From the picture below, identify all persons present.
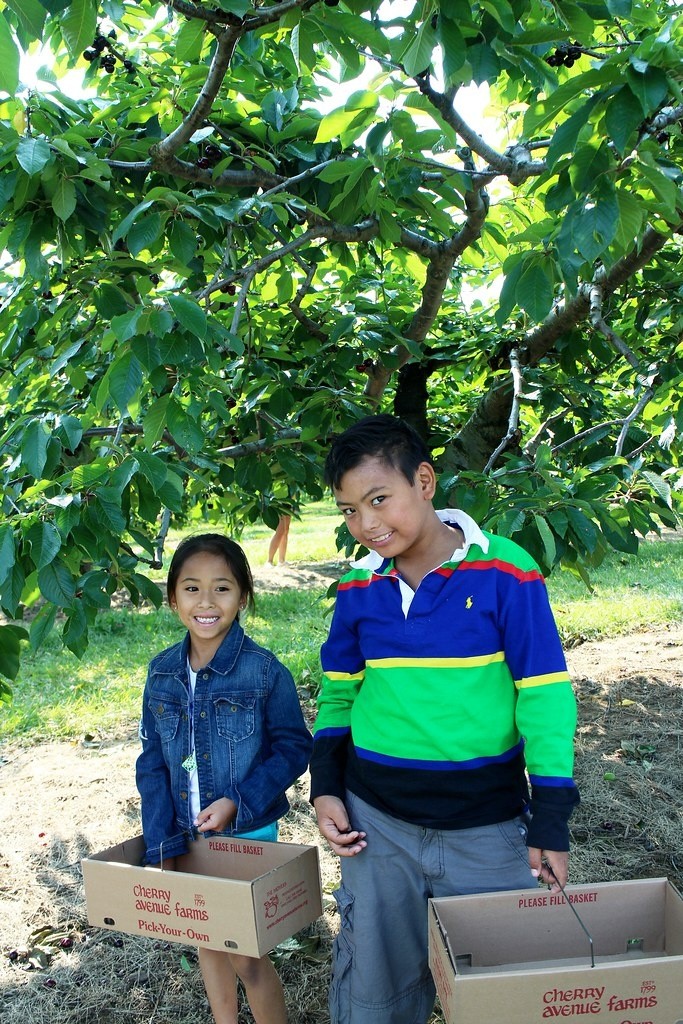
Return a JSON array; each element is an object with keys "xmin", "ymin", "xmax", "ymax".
[
  {"xmin": 135, "ymin": 533, "xmax": 310, "ymax": 1024},
  {"xmin": 268, "ymin": 482, "xmax": 300, "ymax": 567},
  {"xmin": 310, "ymin": 415, "xmax": 581, "ymax": 1023}
]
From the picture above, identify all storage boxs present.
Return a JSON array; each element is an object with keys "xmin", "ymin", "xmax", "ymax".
[
  {"xmin": 428, "ymin": 877, "xmax": 683, "ymax": 1023},
  {"xmin": 81, "ymin": 834, "xmax": 325, "ymax": 959}
]
